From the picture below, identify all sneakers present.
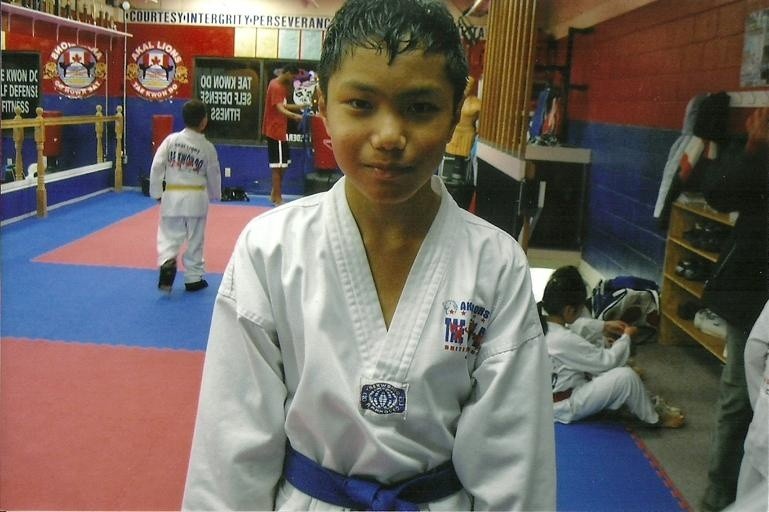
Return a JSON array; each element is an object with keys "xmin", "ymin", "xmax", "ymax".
[
  {"xmin": 159, "ymin": 262, "xmax": 176, "ymax": 291},
  {"xmin": 650, "ymin": 395, "xmax": 684, "ymax": 427},
  {"xmin": 703, "ymin": 479, "xmax": 738, "ymax": 509},
  {"xmin": 674, "ymin": 220, "xmax": 727, "ymax": 340},
  {"xmin": 185, "ymin": 279, "xmax": 209, "ymax": 291}
]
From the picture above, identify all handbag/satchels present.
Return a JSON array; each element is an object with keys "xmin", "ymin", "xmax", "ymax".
[
  {"xmin": 303, "ymin": 147, "xmax": 316, "ymax": 174},
  {"xmin": 585, "ymin": 275, "xmax": 660, "ymax": 345}
]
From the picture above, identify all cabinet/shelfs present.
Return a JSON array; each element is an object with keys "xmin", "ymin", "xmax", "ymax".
[{"xmin": 659, "ymin": 190, "xmax": 737, "ymax": 372}]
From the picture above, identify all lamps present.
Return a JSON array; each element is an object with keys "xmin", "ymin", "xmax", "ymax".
[{"xmin": 117, "ymin": 0, "xmax": 131, "ymax": 12}]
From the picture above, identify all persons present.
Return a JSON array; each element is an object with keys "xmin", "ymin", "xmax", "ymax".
[
  {"xmin": 147, "ymin": 98, "xmax": 223, "ymax": 293},
  {"xmin": 443, "ymin": 74, "xmax": 482, "ymax": 158},
  {"xmin": 700, "ymin": 106, "xmax": 769, "ymax": 510},
  {"xmin": 58, "ymin": 60, "xmax": 173, "ymax": 81},
  {"xmin": 178, "ymin": 0, "xmax": 558, "ymax": 511},
  {"xmin": 722, "ymin": 297, "xmax": 769, "ymax": 511},
  {"xmin": 261, "ymin": 63, "xmax": 308, "ymax": 205},
  {"xmin": 536, "ymin": 265, "xmax": 686, "ymax": 430}
]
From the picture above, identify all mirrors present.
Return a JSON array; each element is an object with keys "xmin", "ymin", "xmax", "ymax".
[{"xmin": 1, "ymin": 8, "xmax": 130, "ymax": 195}]
